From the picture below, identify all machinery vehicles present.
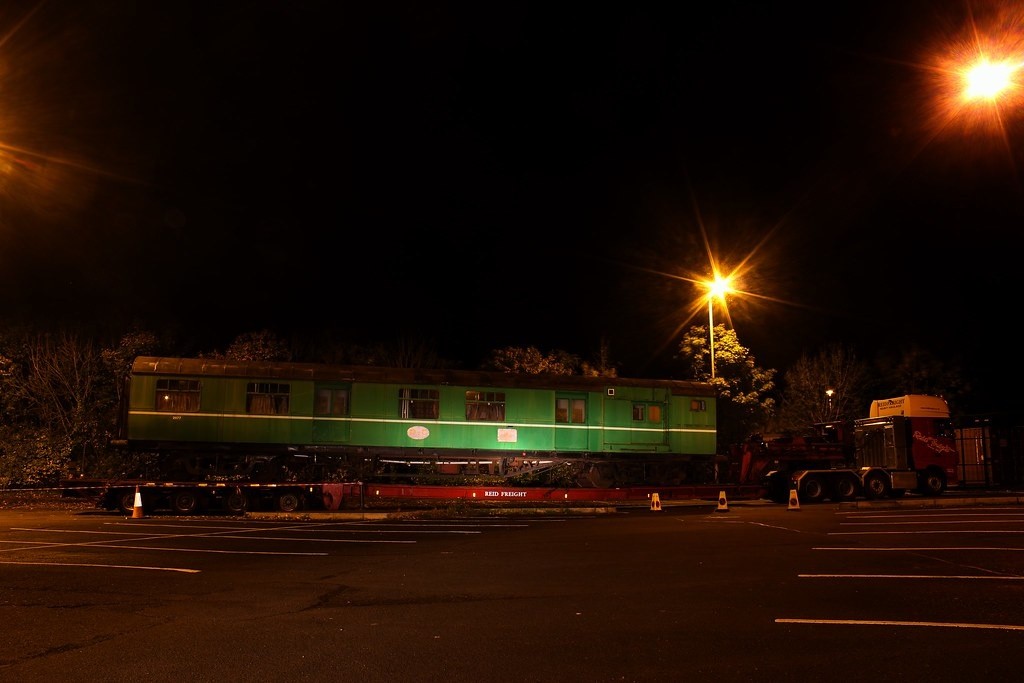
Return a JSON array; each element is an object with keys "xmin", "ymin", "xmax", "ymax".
[{"xmin": 762, "ymin": 421, "xmax": 853, "ymax": 467}]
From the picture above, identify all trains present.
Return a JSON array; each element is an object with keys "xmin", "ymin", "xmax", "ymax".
[{"xmin": 126, "ymin": 355, "xmax": 718, "ymax": 485}]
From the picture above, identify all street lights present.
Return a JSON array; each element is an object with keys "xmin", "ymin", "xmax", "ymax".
[{"xmin": 705, "ymin": 274, "xmax": 734, "ymax": 378}]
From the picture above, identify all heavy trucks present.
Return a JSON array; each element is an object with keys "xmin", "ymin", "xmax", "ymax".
[{"xmin": 766, "ymin": 393, "xmax": 960, "ymax": 499}]
